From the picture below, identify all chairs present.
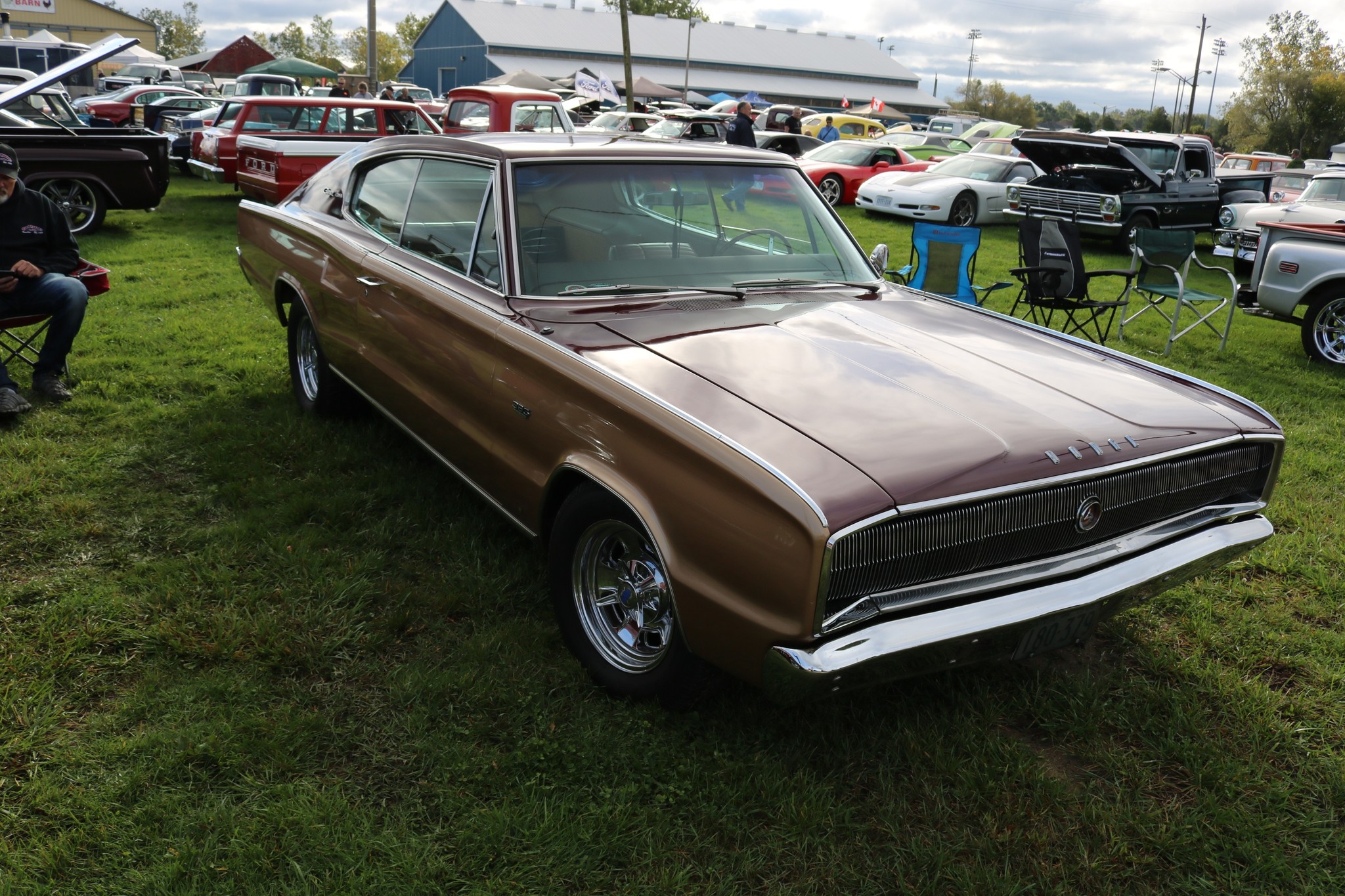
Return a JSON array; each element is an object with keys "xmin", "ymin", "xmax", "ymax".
[
  {"xmin": 879, "ymin": 154, "xmax": 893, "ymax": 165},
  {"xmin": 884, "ymin": 220, "xmax": 1013, "ymax": 307},
  {"xmin": 0, "ymin": 258, "xmax": 111, "ymax": 386},
  {"xmin": 1009, "ymin": 220, "xmax": 1139, "ymax": 348},
  {"xmin": 391, "ymin": 222, "xmax": 483, "ymax": 253},
  {"xmin": 432, "ymin": 250, "xmax": 499, "ymax": 280},
  {"xmin": 608, "ymin": 242, "xmax": 697, "ymax": 260},
  {"xmin": 1118, "ymin": 226, "xmax": 1238, "ymax": 359}
]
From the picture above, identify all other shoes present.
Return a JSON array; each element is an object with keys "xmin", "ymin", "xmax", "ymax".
[
  {"xmin": 32, "ymin": 371, "xmax": 73, "ymax": 402},
  {"xmin": 0, "ymin": 388, "xmax": 31, "ymax": 414}
]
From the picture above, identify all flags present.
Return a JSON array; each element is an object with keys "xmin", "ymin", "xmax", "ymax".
[
  {"xmin": 871, "ymin": 96, "xmax": 885, "ymax": 112},
  {"xmin": 575, "ymin": 71, "xmax": 604, "ymax": 101},
  {"xmin": 840, "ymin": 95, "xmax": 849, "ymax": 108},
  {"xmin": 599, "ymin": 70, "xmax": 622, "ymax": 106}
]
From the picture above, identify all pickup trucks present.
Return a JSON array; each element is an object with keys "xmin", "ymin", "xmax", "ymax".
[
  {"xmin": 1000, "ymin": 132, "xmax": 1277, "ymax": 257},
  {"xmin": 1234, "ymin": 221, "xmax": 1344, "ymax": 368},
  {"xmin": 238, "ymin": 87, "xmax": 584, "ymax": 207}
]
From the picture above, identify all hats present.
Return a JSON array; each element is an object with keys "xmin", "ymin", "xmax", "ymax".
[
  {"xmin": 1288, "ymin": 149, "xmax": 1299, "ymax": 155},
  {"xmin": 0, "ymin": 144, "xmax": 20, "ymax": 179},
  {"xmin": 386, "ymin": 86, "xmax": 393, "ymax": 92}
]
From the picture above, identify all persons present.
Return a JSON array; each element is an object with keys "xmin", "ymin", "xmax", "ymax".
[
  {"xmin": 785, "ymin": 106, "xmax": 840, "ymax": 143},
  {"xmin": 98, "ymin": 68, "xmax": 116, "ymax": 78},
  {"xmin": 1285, "ymin": 149, "xmax": 1305, "ymax": 188},
  {"xmin": 0, "ymin": 143, "xmax": 89, "ymax": 418},
  {"xmin": 721, "ymin": 101, "xmax": 755, "ymax": 211},
  {"xmin": 295, "ymin": 75, "xmax": 415, "ymax": 129},
  {"xmin": 845, "ymin": 110, "xmax": 888, "ymax": 128}
]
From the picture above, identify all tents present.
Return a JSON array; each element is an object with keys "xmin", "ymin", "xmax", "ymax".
[
  {"xmin": 841, "ymin": 103, "xmax": 911, "ymax": 124},
  {"xmin": 479, "ymin": 69, "xmax": 773, "ymax": 111},
  {"xmin": 26, "ymin": 28, "xmax": 65, "ymax": 43},
  {"xmin": 88, "ymin": 33, "xmax": 165, "ymax": 65},
  {"xmin": 244, "ymin": 56, "xmax": 338, "ymax": 87}
]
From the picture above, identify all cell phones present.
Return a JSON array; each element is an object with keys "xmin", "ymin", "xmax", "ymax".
[{"xmin": 0, "ymin": 271, "xmax": 16, "ymax": 279}]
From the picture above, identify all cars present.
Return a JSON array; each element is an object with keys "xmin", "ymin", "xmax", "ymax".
[
  {"xmin": 1213, "ymin": 146, "xmax": 1344, "ymax": 260},
  {"xmin": 0, "ymin": 38, "xmax": 1045, "ymax": 235},
  {"xmin": 235, "ymin": 133, "xmax": 1287, "ymax": 696}
]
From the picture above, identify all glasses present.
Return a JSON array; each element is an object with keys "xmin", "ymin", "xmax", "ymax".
[
  {"xmin": 796, "ymin": 112, "xmax": 802, "ymax": 114},
  {"xmin": 338, "ymin": 82, "xmax": 343, "ymax": 85},
  {"xmin": 827, "ymin": 122, "xmax": 831, "ymax": 123}
]
